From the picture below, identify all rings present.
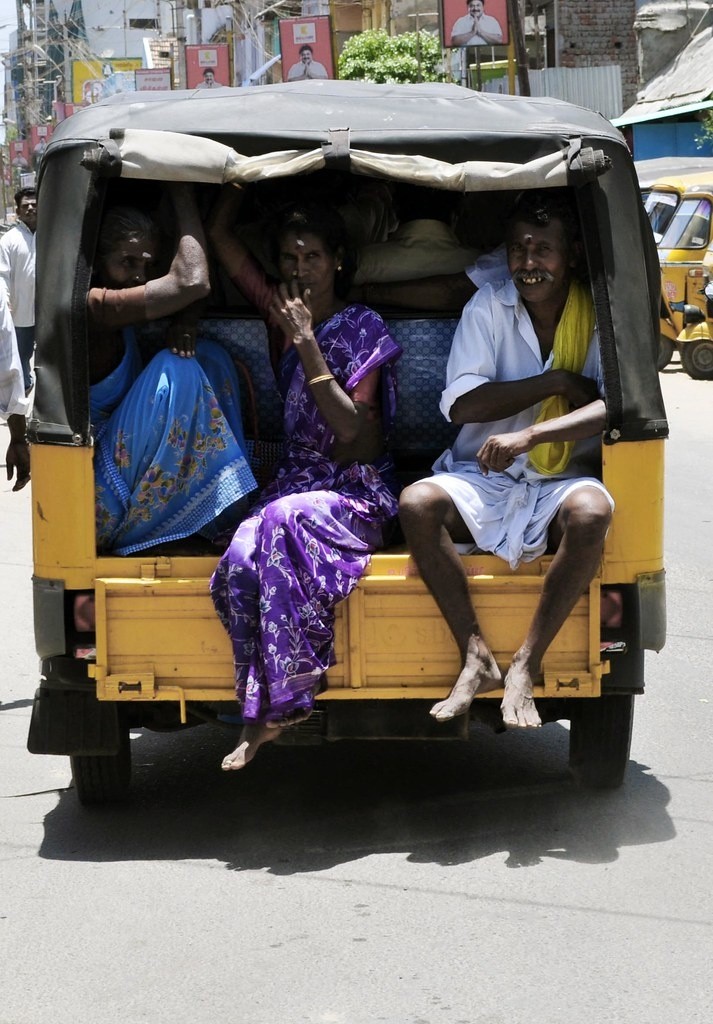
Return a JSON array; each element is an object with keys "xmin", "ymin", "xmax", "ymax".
[{"xmin": 184, "ymin": 333, "xmax": 192, "ymax": 337}]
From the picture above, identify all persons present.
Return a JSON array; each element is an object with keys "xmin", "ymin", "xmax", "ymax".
[
  {"xmin": 12, "ymin": 151, "xmax": 28, "ymax": 165},
  {"xmin": 85, "ymin": 181, "xmax": 259, "ymax": 557},
  {"xmin": 33, "ymin": 136, "xmax": 47, "ymax": 153},
  {"xmin": 344, "ymin": 191, "xmax": 616, "ymax": 730},
  {"xmin": 0, "ymin": 185, "xmax": 38, "ymax": 398},
  {"xmin": 353, "ymin": 182, "xmax": 486, "ymax": 285},
  {"xmin": 450, "ymin": 0, "xmax": 503, "ymax": 46},
  {"xmin": 195, "ymin": 69, "xmax": 223, "ymax": 89},
  {"xmin": 288, "ymin": 45, "xmax": 328, "ymax": 82},
  {"xmin": 209, "ymin": 181, "xmax": 406, "ymax": 771},
  {"xmin": 0, "ymin": 289, "xmax": 32, "ymax": 492}
]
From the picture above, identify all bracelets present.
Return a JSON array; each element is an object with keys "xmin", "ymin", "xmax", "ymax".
[{"xmin": 306, "ymin": 374, "xmax": 335, "ymax": 386}]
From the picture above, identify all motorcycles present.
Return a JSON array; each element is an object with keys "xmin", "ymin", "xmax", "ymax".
[
  {"xmin": 644, "ymin": 169, "xmax": 713, "ymax": 379},
  {"xmin": 24, "ymin": 79, "xmax": 670, "ymax": 810}
]
